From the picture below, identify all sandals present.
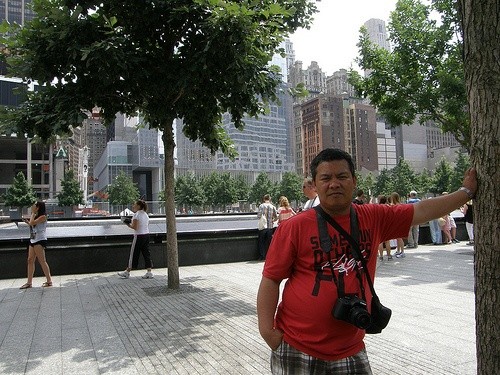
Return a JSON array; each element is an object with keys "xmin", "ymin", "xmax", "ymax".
[
  {"xmin": 42, "ymin": 282, "xmax": 52, "ymax": 287},
  {"xmin": 19, "ymin": 283, "xmax": 32, "ymax": 289}
]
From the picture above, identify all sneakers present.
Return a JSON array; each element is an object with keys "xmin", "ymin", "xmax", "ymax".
[
  {"xmin": 118, "ymin": 270, "xmax": 130, "ymax": 279},
  {"xmin": 141, "ymin": 272, "xmax": 152, "ymax": 279}
]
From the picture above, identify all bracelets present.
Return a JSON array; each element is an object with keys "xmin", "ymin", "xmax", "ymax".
[{"xmin": 459, "ymin": 188, "xmax": 474, "ymax": 200}]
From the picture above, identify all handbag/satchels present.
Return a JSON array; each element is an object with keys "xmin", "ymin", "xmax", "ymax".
[{"xmin": 258, "ymin": 202, "xmax": 267, "ymax": 230}]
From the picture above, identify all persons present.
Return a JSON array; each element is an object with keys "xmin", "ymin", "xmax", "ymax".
[
  {"xmin": 118, "ymin": 200, "xmax": 154, "ymax": 278},
  {"xmin": 176, "ymin": 208, "xmax": 194, "ymax": 216},
  {"xmin": 255, "ymin": 194, "xmax": 278, "ymax": 261},
  {"xmin": 277, "ymin": 196, "xmax": 297, "ymax": 224},
  {"xmin": 292, "ymin": 205, "xmax": 304, "ymax": 214},
  {"xmin": 302, "ymin": 177, "xmax": 321, "ymax": 211},
  {"xmin": 352, "ymin": 190, "xmax": 474, "ymax": 260},
  {"xmin": 20, "ymin": 201, "xmax": 52, "ymax": 288},
  {"xmin": 256, "ymin": 148, "xmax": 478, "ymax": 375}
]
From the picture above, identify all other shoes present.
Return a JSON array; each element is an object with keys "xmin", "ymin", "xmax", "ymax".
[
  {"xmin": 466, "ymin": 241, "xmax": 474, "ymax": 246},
  {"xmin": 452, "ymin": 238, "xmax": 460, "ymax": 244},
  {"xmin": 443, "ymin": 241, "xmax": 448, "ymax": 244},
  {"xmin": 447, "ymin": 241, "xmax": 451, "ymax": 244},
  {"xmin": 433, "ymin": 242, "xmax": 442, "ymax": 245},
  {"xmin": 392, "ymin": 251, "xmax": 402, "ymax": 258},
  {"xmin": 400, "ymin": 252, "xmax": 405, "ymax": 258},
  {"xmin": 406, "ymin": 243, "xmax": 414, "ymax": 248},
  {"xmin": 388, "ymin": 256, "xmax": 393, "ymax": 261},
  {"xmin": 377, "ymin": 256, "xmax": 383, "ymax": 261},
  {"xmin": 414, "ymin": 243, "xmax": 418, "ymax": 248}
]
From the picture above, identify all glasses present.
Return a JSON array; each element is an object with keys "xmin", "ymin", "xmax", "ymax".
[{"xmin": 303, "ymin": 185, "xmax": 314, "ymax": 189}]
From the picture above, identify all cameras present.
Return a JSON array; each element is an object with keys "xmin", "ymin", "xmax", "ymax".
[{"xmin": 333, "ymin": 296, "xmax": 371, "ymax": 328}]
